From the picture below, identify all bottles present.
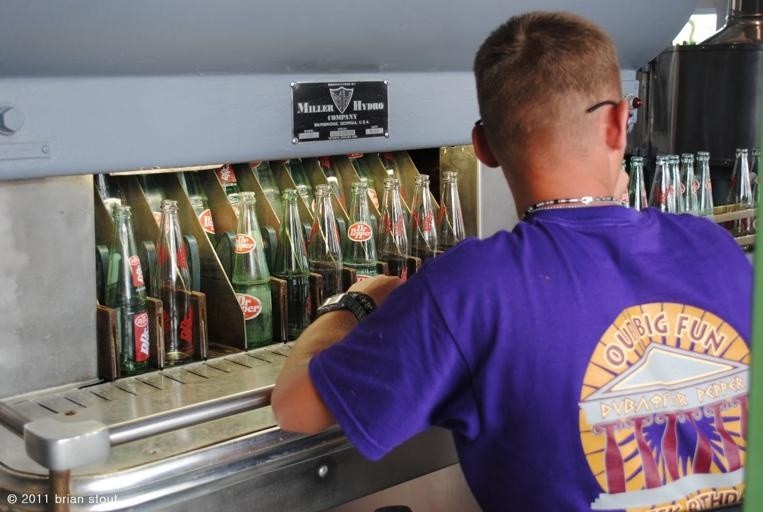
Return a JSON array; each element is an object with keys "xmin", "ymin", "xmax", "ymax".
[
  {"xmin": 377, "ymin": 152, "xmax": 406, "ymax": 186},
  {"xmin": 105, "ymin": 201, "xmax": 152, "ymax": 373},
  {"xmin": 184, "ymin": 170, "xmax": 221, "ymax": 244},
  {"xmin": 319, "ymin": 160, "xmax": 344, "ymax": 199},
  {"xmin": 680, "ymin": 152, "xmax": 698, "ymax": 212},
  {"xmin": 619, "ymin": 160, "xmax": 631, "ymax": 208},
  {"xmin": 155, "ymin": 197, "xmax": 198, "ymax": 362},
  {"xmin": 381, "ymin": 177, "xmax": 410, "ymax": 279},
  {"xmin": 277, "ymin": 187, "xmax": 314, "ymax": 342},
  {"xmin": 235, "ymin": 192, "xmax": 275, "ymax": 344},
  {"xmin": 727, "ymin": 147, "xmax": 753, "ymax": 251},
  {"xmin": 350, "ymin": 182, "xmax": 380, "ymax": 280},
  {"xmin": 215, "ymin": 166, "xmax": 241, "ymax": 198},
  {"xmin": 695, "ymin": 151, "xmax": 714, "ymax": 214},
  {"xmin": 437, "ymin": 171, "xmax": 467, "ymax": 252},
  {"xmin": 98, "ymin": 173, "xmax": 138, "ymax": 235},
  {"xmin": 649, "ymin": 156, "xmax": 671, "ymax": 212},
  {"xmin": 144, "ymin": 172, "xmax": 181, "ymax": 226},
  {"xmin": 669, "ymin": 155, "xmax": 682, "ymax": 213},
  {"xmin": 629, "ymin": 156, "xmax": 650, "ymax": 210},
  {"xmin": 286, "ymin": 160, "xmax": 313, "ymax": 205},
  {"xmin": 313, "ymin": 183, "xmax": 345, "ymax": 305},
  {"xmin": 348, "ymin": 154, "xmax": 375, "ymax": 189},
  {"xmin": 412, "ymin": 175, "xmax": 435, "ymax": 265},
  {"xmin": 249, "ymin": 160, "xmax": 283, "ymax": 218}
]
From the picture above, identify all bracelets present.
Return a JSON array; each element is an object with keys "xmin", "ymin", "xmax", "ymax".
[{"xmin": 348, "ymin": 292, "xmax": 378, "ymax": 315}]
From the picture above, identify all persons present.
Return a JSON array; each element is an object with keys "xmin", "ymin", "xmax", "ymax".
[{"xmin": 268, "ymin": 10, "xmax": 754, "ymax": 512}]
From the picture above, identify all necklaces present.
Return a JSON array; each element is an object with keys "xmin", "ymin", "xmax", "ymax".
[{"xmin": 519, "ymin": 196, "xmax": 626, "ymax": 215}]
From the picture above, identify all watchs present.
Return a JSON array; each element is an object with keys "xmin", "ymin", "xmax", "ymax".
[{"xmin": 315, "ymin": 293, "xmax": 368, "ymax": 324}]
